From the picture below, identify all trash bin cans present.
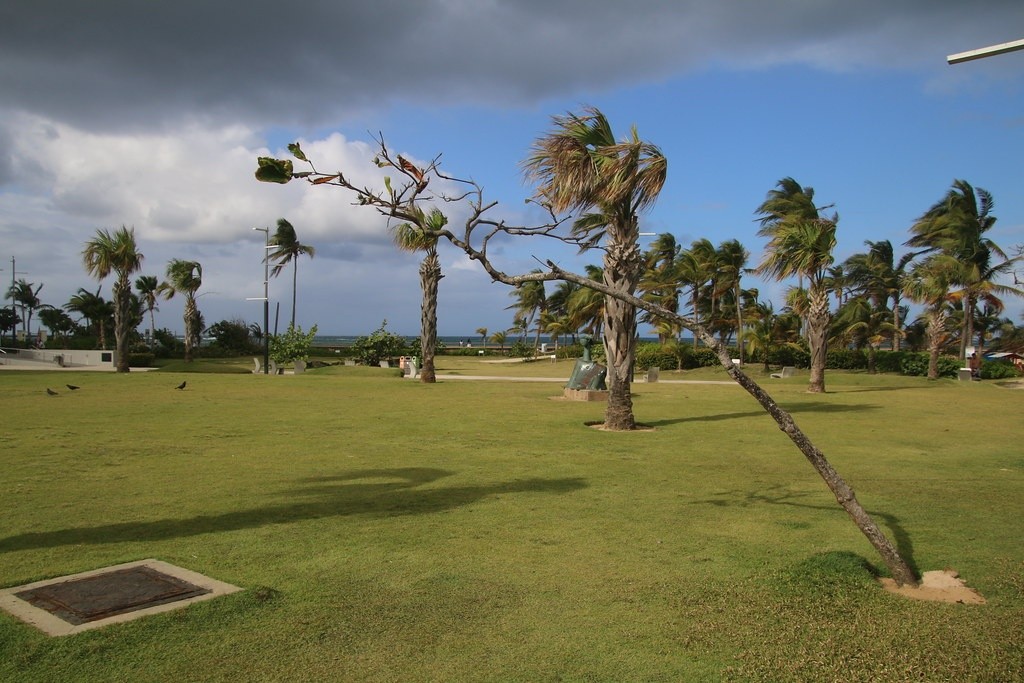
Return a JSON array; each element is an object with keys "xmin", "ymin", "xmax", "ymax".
[
  {"xmin": 399, "ymin": 356, "xmax": 419, "ymax": 375},
  {"xmin": 541, "ymin": 343, "xmax": 547, "ymax": 352}
]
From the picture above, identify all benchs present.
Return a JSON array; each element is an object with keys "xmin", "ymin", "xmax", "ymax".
[{"xmin": 771, "ymin": 367, "xmax": 795, "ymax": 379}]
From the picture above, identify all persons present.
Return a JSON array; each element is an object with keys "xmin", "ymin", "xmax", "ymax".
[
  {"xmin": 467, "ymin": 339, "xmax": 471, "ymax": 347},
  {"xmin": 459, "ymin": 340, "xmax": 463, "ymax": 346},
  {"xmin": 37, "ymin": 338, "xmax": 45, "ymax": 349}
]
